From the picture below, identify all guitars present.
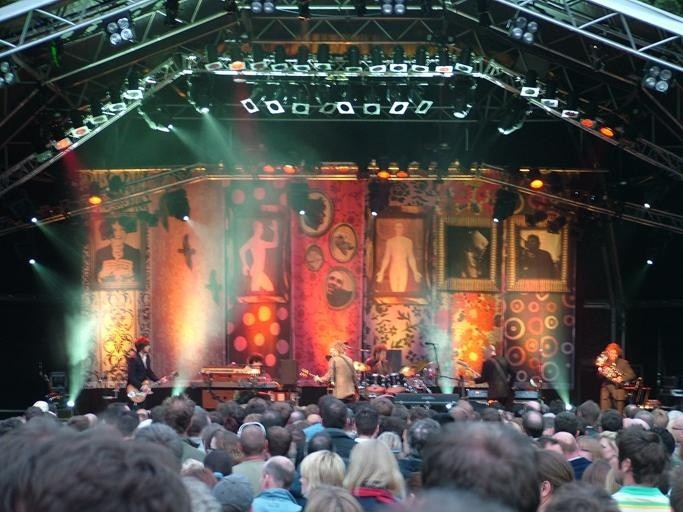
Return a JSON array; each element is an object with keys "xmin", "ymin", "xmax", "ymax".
[
  {"xmin": 127, "ymin": 371, "xmax": 180, "ymax": 404},
  {"xmin": 300, "ymin": 368, "xmax": 359, "ymax": 404}
]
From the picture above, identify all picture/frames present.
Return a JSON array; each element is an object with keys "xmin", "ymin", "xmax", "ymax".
[
  {"xmin": 89, "ymin": 210, "xmax": 148, "ymax": 290},
  {"xmin": 435, "ymin": 214, "xmax": 497, "ymax": 292},
  {"xmin": 505, "ymin": 214, "xmax": 568, "ymax": 294}
]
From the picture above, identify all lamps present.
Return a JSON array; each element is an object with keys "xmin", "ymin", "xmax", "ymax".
[
  {"xmin": 497, "ymin": 70, "xmax": 639, "ymax": 139},
  {"xmin": 366, "ymin": 178, "xmax": 392, "ymax": 216},
  {"xmin": 0, "ymin": 62, "xmax": 19, "ymax": 89},
  {"xmin": 508, "ymin": 15, "xmax": 539, "ymax": 42},
  {"xmin": 31, "ymin": 66, "xmax": 211, "ymax": 161},
  {"xmin": 524, "ymin": 199, "xmax": 568, "ymax": 236},
  {"xmin": 234, "ymin": 78, "xmax": 477, "ymax": 118},
  {"xmin": 186, "ymin": 40, "xmax": 474, "ymax": 78},
  {"xmin": 163, "ymin": 0, "xmax": 179, "ymax": 26},
  {"xmin": 488, "ymin": 190, "xmax": 521, "ymax": 225},
  {"xmin": 158, "ymin": 188, "xmax": 194, "ymax": 226},
  {"xmin": 252, "ymin": 0, "xmax": 437, "ymax": 20},
  {"xmin": 105, "ymin": 11, "xmax": 136, "ymax": 48},
  {"xmin": 643, "ymin": 65, "xmax": 674, "ymax": 93},
  {"xmin": 284, "ymin": 182, "xmax": 312, "ymax": 215},
  {"xmin": 96, "ymin": 200, "xmax": 158, "ymax": 241},
  {"xmin": 86, "ymin": 179, "xmax": 104, "ymax": 206}
]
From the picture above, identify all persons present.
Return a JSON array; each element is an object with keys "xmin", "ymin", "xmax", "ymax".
[
  {"xmin": 94, "ymin": 217, "xmax": 141, "ymax": 288},
  {"xmin": 376, "ymin": 222, "xmax": 423, "ymax": 293},
  {"xmin": 524, "ymin": 235, "xmax": 554, "ymax": 279},
  {"xmin": 239, "ymin": 219, "xmax": 279, "ymax": 291}
]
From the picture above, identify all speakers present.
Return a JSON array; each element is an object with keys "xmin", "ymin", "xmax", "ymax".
[
  {"xmin": 279, "ymin": 359, "xmax": 296, "ymax": 384},
  {"xmin": 394, "ymin": 392, "xmax": 460, "ymax": 404},
  {"xmin": 1, "ymin": 409, "xmax": 74, "ymax": 418},
  {"xmin": 386, "ymin": 349, "xmax": 402, "ymax": 370}
]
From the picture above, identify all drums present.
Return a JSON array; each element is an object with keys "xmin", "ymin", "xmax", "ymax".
[
  {"xmin": 385, "ymin": 373, "xmax": 406, "ymax": 393},
  {"xmin": 366, "ymin": 374, "xmax": 386, "ymax": 391}
]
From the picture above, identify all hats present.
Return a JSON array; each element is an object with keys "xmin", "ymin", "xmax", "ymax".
[{"xmin": 134, "ymin": 336, "xmax": 151, "ymax": 352}]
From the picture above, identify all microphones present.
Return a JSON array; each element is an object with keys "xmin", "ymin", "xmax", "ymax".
[
  {"xmin": 424, "ymin": 341, "xmax": 434, "ymax": 345},
  {"xmin": 539, "ymin": 342, "xmax": 544, "ymax": 352}
]
